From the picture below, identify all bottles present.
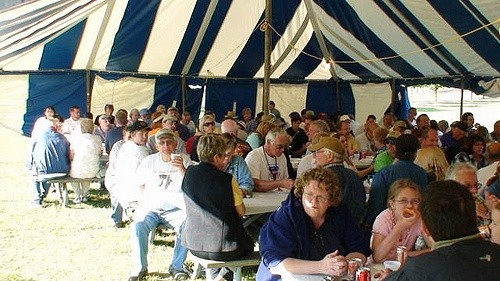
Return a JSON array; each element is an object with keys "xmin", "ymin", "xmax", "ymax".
[{"xmin": 415, "ymin": 235, "xmax": 429, "ymax": 252}]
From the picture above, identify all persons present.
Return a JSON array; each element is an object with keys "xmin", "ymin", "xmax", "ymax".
[
  {"xmin": 127, "ymin": 128, "xmax": 195, "ymax": 281},
  {"xmin": 256, "ymin": 165, "xmax": 370, "ymax": 281},
  {"xmin": 379, "ymin": 180, "xmax": 500, "ymax": 281},
  {"xmin": 26, "ymin": 101, "xmax": 500, "ymax": 245},
  {"xmin": 371, "ymin": 178, "xmax": 435, "ymax": 263},
  {"xmin": 181, "ymin": 133, "xmax": 256, "ymax": 281}
]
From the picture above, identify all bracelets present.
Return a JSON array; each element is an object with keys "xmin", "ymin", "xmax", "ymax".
[{"xmin": 394, "ymin": 226, "xmax": 402, "ymax": 240}]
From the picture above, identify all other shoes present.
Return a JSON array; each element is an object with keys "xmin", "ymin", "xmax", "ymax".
[
  {"xmin": 169, "ymin": 264, "xmax": 188, "ymax": 281},
  {"xmin": 72, "ymin": 197, "xmax": 81, "ymax": 203},
  {"xmin": 115, "ymin": 222, "xmax": 123, "ymax": 228},
  {"xmin": 80, "ymin": 196, "xmax": 87, "ymax": 202},
  {"xmin": 35, "ymin": 193, "xmax": 45, "ymax": 204},
  {"xmin": 128, "ymin": 269, "xmax": 149, "ymax": 281}
]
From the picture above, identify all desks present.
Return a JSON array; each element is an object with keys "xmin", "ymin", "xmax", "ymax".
[
  {"xmin": 280, "ymin": 263, "xmax": 383, "ymax": 281},
  {"xmin": 241, "ymin": 191, "xmax": 289, "ymax": 215},
  {"xmin": 354, "ymin": 158, "xmax": 373, "ymax": 168}
]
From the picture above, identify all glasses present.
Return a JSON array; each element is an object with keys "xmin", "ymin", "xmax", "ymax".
[
  {"xmin": 463, "ymin": 182, "xmax": 482, "ymax": 190},
  {"xmin": 392, "ymin": 198, "xmax": 421, "ymax": 208},
  {"xmin": 313, "ymin": 149, "xmax": 327, "ymax": 159},
  {"xmin": 397, "ymin": 125, "xmax": 406, "ymax": 131},
  {"xmin": 385, "ymin": 139, "xmax": 395, "ymax": 145},
  {"xmin": 204, "ymin": 121, "xmax": 215, "ymax": 127},
  {"xmin": 157, "ymin": 140, "xmax": 176, "ymax": 146},
  {"xmin": 270, "ymin": 140, "xmax": 288, "ymax": 150}
]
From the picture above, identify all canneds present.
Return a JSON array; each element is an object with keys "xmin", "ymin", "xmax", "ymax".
[
  {"xmin": 397, "ymin": 246, "xmax": 408, "ymax": 265},
  {"xmin": 361, "ymin": 151, "xmax": 366, "ymax": 158},
  {"xmin": 347, "ymin": 258, "xmax": 371, "ymax": 281}
]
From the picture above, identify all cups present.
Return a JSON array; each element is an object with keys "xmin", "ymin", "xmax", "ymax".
[
  {"xmin": 384, "ymin": 261, "xmax": 402, "ymax": 271},
  {"xmin": 354, "ymin": 154, "xmax": 360, "ymax": 160},
  {"xmin": 171, "ymin": 154, "xmax": 180, "ymax": 162}
]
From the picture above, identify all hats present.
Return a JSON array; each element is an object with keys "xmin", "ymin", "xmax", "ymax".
[
  {"xmin": 306, "ymin": 137, "xmax": 346, "ymax": 156},
  {"xmin": 155, "ymin": 128, "xmax": 176, "ymax": 142},
  {"xmin": 131, "ymin": 121, "xmax": 152, "ymax": 132},
  {"xmin": 162, "ymin": 115, "xmax": 178, "ymax": 124},
  {"xmin": 385, "ymin": 131, "xmax": 401, "ymax": 138},
  {"xmin": 340, "ymin": 115, "xmax": 351, "ymax": 122}
]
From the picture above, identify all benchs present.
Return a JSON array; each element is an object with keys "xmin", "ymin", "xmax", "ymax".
[
  {"xmin": 197, "ymin": 251, "xmax": 261, "ymax": 281},
  {"xmin": 47, "ymin": 177, "xmax": 105, "ymax": 209}
]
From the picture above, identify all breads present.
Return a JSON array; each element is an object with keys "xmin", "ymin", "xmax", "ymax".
[{"xmin": 404, "ymin": 208, "xmax": 415, "ymax": 215}]
